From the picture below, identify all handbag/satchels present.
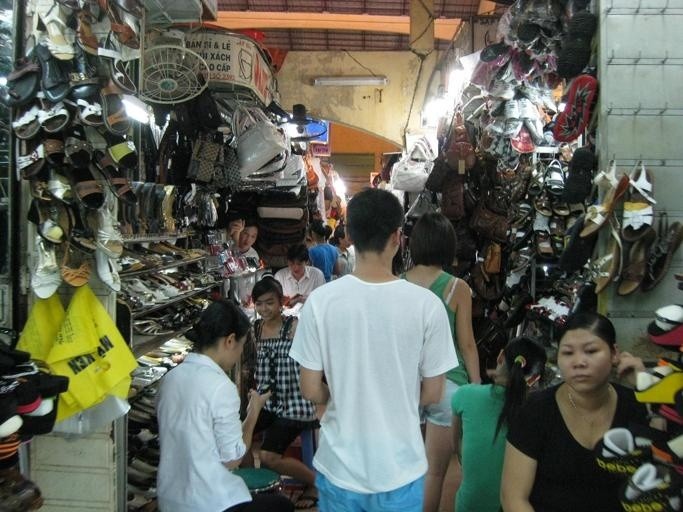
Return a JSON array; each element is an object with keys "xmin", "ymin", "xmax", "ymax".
[
  {"xmin": 428, "ymin": 103, "xmax": 478, "ymax": 222},
  {"xmin": 226, "ymin": 102, "xmax": 307, "ymax": 186},
  {"xmin": 391, "ymin": 136, "xmax": 433, "ymax": 193},
  {"xmin": 185, "ymin": 130, "xmax": 240, "ymax": 187}
]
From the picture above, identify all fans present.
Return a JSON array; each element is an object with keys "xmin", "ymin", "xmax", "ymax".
[{"xmin": 138, "ymin": 30, "xmax": 213, "ymax": 106}]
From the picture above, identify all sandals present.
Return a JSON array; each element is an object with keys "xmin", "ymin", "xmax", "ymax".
[
  {"xmin": 534, "ymin": 189, "xmax": 553, "ymax": 218},
  {"xmin": 546, "ymin": 159, "xmax": 566, "ymax": 194},
  {"xmin": 148, "ymin": 313, "xmax": 174, "ymax": 331},
  {"xmin": 36, "ymin": 92, "xmax": 69, "ymax": 133},
  {"xmin": 515, "ymin": 198, "xmax": 534, "ymax": 223},
  {"xmin": 100, "ymin": 84, "xmax": 131, "ymax": 135},
  {"xmin": 495, "ymin": 289, "xmax": 522, "ymax": 320},
  {"xmin": 76, "ymin": 95, "xmax": 105, "ymax": 126},
  {"xmin": 48, "ymin": 165, "xmax": 75, "ymax": 207},
  {"xmin": 588, "ymin": 209, "xmax": 623, "ymax": 296},
  {"xmin": 133, "ymin": 318, "xmax": 174, "ymax": 336},
  {"xmin": 66, "ymin": 51, "xmax": 101, "ymax": 100},
  {"xmin": 551, "ymin": 196, "xmax": 571, "ymax": 216},
  {"xmin": 578, "ymin": 159, "xmax": 630, "ymax": 241},
  {"xmin": 641, "ymin": 218, "xmax": 683, "ymax": 292},
  {"xmin": 533, "ymin": 211, "xmax": 550, "ymax": 237},
  {"xmin": 116, "ymin": 259, "xmax": 215, "ymax": 310},
  {"xmin": 191, "ymin": 297, "xmax": 209, "ymax": 309},
  {"xmin": 513, "ymin": 217, "xmax": 533, "ymax": 249},
  {"xmin": 32, "ymin": 235, "xmax": 64, "ymax": 300},
  {"xmin": 38, "ymin": 47, "xmax": 70, "ymax": 103},
  {"xmin": 172, "ymin": 304, "xmax": 201, "ymax": 330},
  {"xmin": 91, "ymin": 148, "xmax": 138, "ymax": 208},
  {"xmin": 537, "ymin": 237, "xmax": 554, "ymax": 258},
  {"xmin": 96, "ymin": 197, "xmax": 124, "ymax": 259},
  {"xmin": 62, "ymin": 245, "xmax": 91, "ymax": 287},
  {"xmin": 59, "ymin": 199, "xmax": 98, "ymax": 256},
  {"xmin": 616, "ymin": 225, "xmax": 655, "ymax": 299},
  {"xmin": 552, "ymin": 234, "xmax": 564, "ymax": 259},
  {"xmin": 528, "ymin": 159, "xmax": 546, "ymax": 196},
  {"xmin": 504, "ymin": 294, "xmax": 532, "ymax": 328},
  {"xmin": 9, "ymin": 102, "xmax": 39, "ymax": 138},
  {"xmin": 623, "ymin": 158, "xmax": 654, "ymax": 239},
  {"xmin": 26, "ymin": 174, "xmax": 53, "ymax": 202}
]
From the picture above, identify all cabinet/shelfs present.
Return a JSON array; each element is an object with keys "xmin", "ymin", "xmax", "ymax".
[
  {"xmin": 0, "ymin": 0, "xmax": 45, "ymax": 477},
  {"xmin": 18, "ymin": 0, "xmax": 240, "ymax": 512}
]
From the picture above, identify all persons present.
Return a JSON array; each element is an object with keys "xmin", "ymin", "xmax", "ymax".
[
  {"xmin": 307, "ymin": 219, "xmax": 341, "ymax": 282},
  {"xmin": 231, "ymin": 277, "xmax": 327, "ymax": 509},
  {"xmin": 394, "ymin": 212, "xmax": 481, "ymax": 511},
  {"xmin": 274, "ymin": 245, "xmax": 326, "ymax": 318},
  {"xmin": 289, "ymin": 190, "xmax": 454, "ymax": 512},
  {"xmin": 450, "ymin": 339, "xmax": 547, "ymax": 512},
  {"xmin": 157, "ymin": 300, "xmax": 293, "ymax": 512},
  {"xmin": 221, "ymin": 218, "xmax": 264, "ymax": 312},
  {"xmin": 498, "ymin": 312, "xmax": 657, "ymax": 512},
  {"xmin": 329, "ymin": 236, "xmax": 338, "ymax": 246},
  {"xmin": 334, "ymin": 224, "xmax": 354, "ymax": 278}
]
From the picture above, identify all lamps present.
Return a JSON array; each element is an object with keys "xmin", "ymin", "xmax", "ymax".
[{"xmin": 311, "ymin": 74, "xmax": 388, "ymax": 89}]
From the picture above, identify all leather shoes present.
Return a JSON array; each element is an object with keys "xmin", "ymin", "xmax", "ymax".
[
  {"xmin": 97, "ymin": 240, "xmax": 122, "ymax": 292},
  {"xmin": 123, "ymin": 182, "xmax": 183, "ymax": 234},
  {"xmin": 128, "ymin": 495, "xmax": 150, "ymax": 510},
  {"xmin": 130, "ymin": 338, "xmax": 192, "ymax": 388},
  {"xmin": 129, "ymin": 457, "xmax": 159, "ymax": 475},
  {"xmin": 126, "ymin": 465, "xmax": 155, "ymax": 484},
  {"xmin": 117, "ymin": 241, "xmax": 206, "ymax": 274},
  {"xmin": 128, "ymin": 482, "xmax": 159, "ymax": 500}
]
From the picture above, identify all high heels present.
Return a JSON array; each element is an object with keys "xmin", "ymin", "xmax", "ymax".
[
  {"xmin": 32, "ymin": 2, "xmax": 75, "ymax": 60},
  {"xmin": 73, "ymin": 3, "xmax": 100, "ymax": 57},
  {"xmin": 26, "ymin": 200, "xmax": 66, "ymax": 244},
  {"xmin": 99, "ymin": 0, "xmax": 139, "ymax": 51}
]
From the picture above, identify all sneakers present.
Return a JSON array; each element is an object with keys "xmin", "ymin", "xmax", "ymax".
[
  {"xmin": 565, "ymin": 147, "xmax": 599, "ymax": 205},
  {"xmin": 557, "ymin": 10, "xmax": 598, "ymax": 80},
  {"xmin": 478, "ymin": 9, "xmax": 553, "ymax": 164},
  {"xmin": 510, "ymin": 245, "xmax": 537, "ymax": 274},
  {"xmin": 552, "ymin": 76, "xmax": 596, "ymax": 143}
]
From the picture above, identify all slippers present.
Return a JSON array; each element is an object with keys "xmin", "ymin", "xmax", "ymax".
[
  {"xmin": 6, "ymin": 48, "xmax": 40, "ymax": 107},
  {"xmin": 65, "ymin": 125, "xmax": 92, "ymax": 169},
  {"xmin": 0, "ymin": 336, "xmax": 70, "ymax": 446},
  {"xmin": 15, "ymin": 142, "xmax": 45, "ymax": 180},
  {"xmin": 45, "ymin": 138, "xmax": 63, "ymax": 166},
  {"xmin": 293, "ymin": 493, "xmax": 318, "ymax": 510},
  {"xmin": 104, "ymin": 129, "xmax": 138, "ymax": 170},
  {"xmin": 74, "ymin": 166, "xmax": 106, "ymax": 211}
]
